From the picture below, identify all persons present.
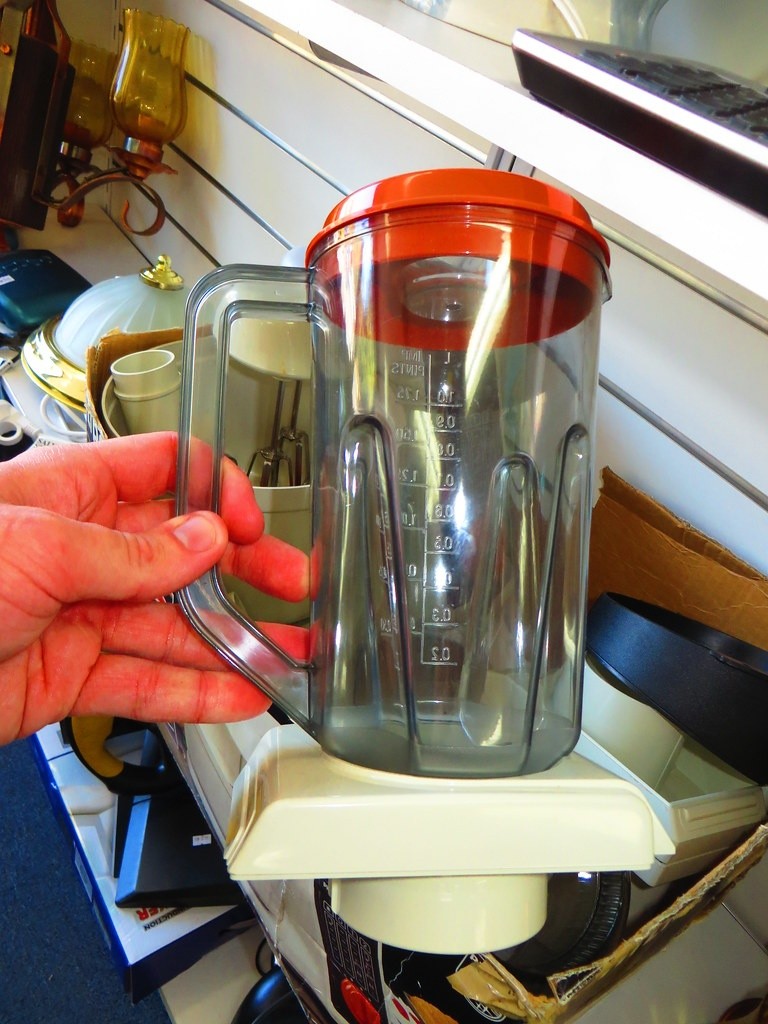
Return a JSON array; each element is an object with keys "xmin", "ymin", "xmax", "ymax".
[{"xmin": 0, "ymin": 431, "xmax": 312, "ymax": 752}]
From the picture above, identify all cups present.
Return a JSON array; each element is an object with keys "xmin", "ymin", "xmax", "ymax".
[{"xmin": 111, "ymin": 350, "xmax": 180, "ymax": 435}]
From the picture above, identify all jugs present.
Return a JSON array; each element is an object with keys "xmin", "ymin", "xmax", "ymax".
[{"xmin": 173, "ymin": 167, "xmax": 613, "ymax": 782}]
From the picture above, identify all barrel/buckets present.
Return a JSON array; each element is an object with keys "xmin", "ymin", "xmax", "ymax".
[{"xmin": 100, "ymin": 334, "xmax": 311, "ymax": 625}]
